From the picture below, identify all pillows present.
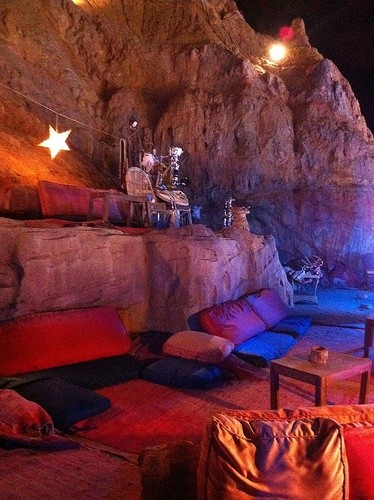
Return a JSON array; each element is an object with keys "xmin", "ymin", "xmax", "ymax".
[
  {"xmin": 11, "ymin": 380, "xmax": 109, "ymax": 429},
  {"xmin": 164, "ymin": 330, "xmax": 235, "ymax": 363},
  {"xmin": 138, "ymin": 357, "xmax": 226, "ymax": 390},
  {"xmin": 0, "ymin": 389, "xmax": 84, "ymax": 450}
]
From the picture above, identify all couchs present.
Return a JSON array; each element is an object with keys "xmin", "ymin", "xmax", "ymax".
[
  {"xmin": 188, "ymin": 287, "xmax": 312, "ymax": 367},
  {"xmin": 196, "ymin": 407, "xmax": 374, "ymax": 500}
]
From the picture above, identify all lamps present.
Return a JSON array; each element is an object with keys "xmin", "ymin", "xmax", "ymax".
[
  {"xmin": 37, "ymin": 114, "xmax": 73, "ymax": 160},
  {"xmin": 129, "ymin": 116, "xmax": 138, "ymax": 128}
]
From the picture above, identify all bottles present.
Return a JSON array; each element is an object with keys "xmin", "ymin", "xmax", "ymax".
[{"xmin": 153, "ymin": 211, "xmax": 163, "ymax": 229}]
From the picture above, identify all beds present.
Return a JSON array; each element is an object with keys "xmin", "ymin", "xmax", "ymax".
[{"xmin": 0, "ymin": 305, "xmax": 230, "ymax": 500}]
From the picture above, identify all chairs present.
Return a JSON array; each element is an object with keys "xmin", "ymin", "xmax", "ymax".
[{"xmin": 126, "ymin": 167, "xmax": 177, "ymax": 230}]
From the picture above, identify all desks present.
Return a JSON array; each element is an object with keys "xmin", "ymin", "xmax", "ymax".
[
  {"xmin": 287, "ymin": 274, "xmax": 319, "ymax": 305},
  {"xmin": 270, "ymin": 349, "xmax": 372, "ymax": 410},
  {"xmin": 88, "ymin": 192, "xmax": 153, "ymax": 229}
]
